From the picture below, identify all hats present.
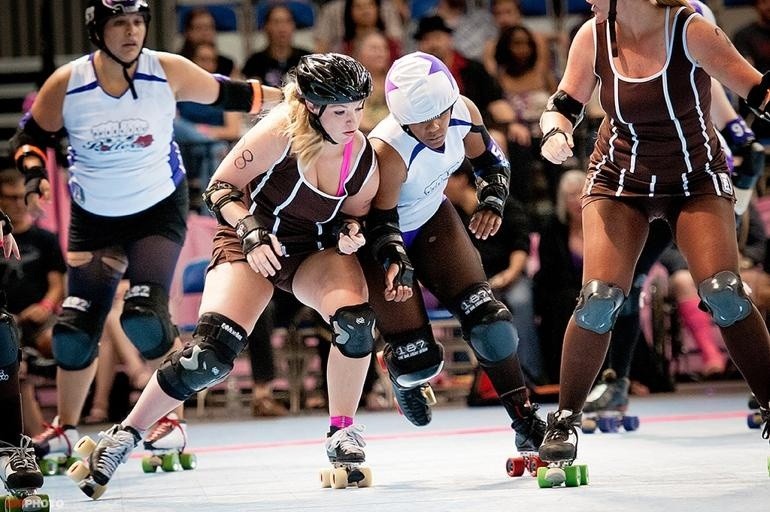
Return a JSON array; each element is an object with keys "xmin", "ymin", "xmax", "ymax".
[{"xmin": 411, "ymin": 15, "xmax": 454, "ymax": 40}]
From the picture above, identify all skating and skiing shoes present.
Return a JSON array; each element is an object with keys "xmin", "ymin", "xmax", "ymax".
[
  {"xmin": 506, "ymin": 403, "xmax": 549, "ymax": 477},
  {"xmin": 143, "ymin": 413, "xmax": 196, "ymax": 473},
  {"xmin": 0, "ymin": 435, "xmax": 50, "ymax": 512},
  {"xmin": 320, "ymin": 424, "xmax": 371, "ymax": 488},
  {"xmin": 581, "ymin": 370, "xmax": 639, "ymax": 434},
  {"xmin": 537, "ymin": 408, "xmax": 588, "ymax": 488},
  {"xmin": 747, "ymin": 391, "xmax": 760, "ymax": 429},
  {"xmin": 65, "ymin": 424, "xmax": 142, "ymax": 500},
  {"xmin": 31, "ymin": 416, "xmax": 80, "ymax": 476},
  {"xmin": 376, "ymin": 342, "xmax": 432, "ymax": 426}
]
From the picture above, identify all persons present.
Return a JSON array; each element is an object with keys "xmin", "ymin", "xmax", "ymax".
[
  {"xmin": 348, "ymin": 34, "xmax": 391, "ymax": 131},
  {"xmin": 0, "ymin": 168, "xmax": 67, "ymax": 436},
  {"xmin": 562, "ymin": 166, "xmax": 591, "ymax": 266},
  {"xmin": 432, "ymin": 1, "xmax": 467, "ymax": 31},
  {"xmin": 356, "ymin": 51, "xmax": 549, "ymax": 477},
  {"xmin": 69, "ymin": 53, "xmax": 380, "ymax": 501},
  {"xmin": 0, "ymin": 207, "xmax": 50, "ymax": 512},
  {"xmin": 495, "ymin": 25, "xmax": 558, "ymax": 137},
  {"xmin": 83, "ymin": 278, "xmax": 155, "ymax": 427},
  {"xmin": 9, "ymin": 1, "xmax": 284, "ymax": 476},
  {"xmin": 576, "ymin": 1, "xmax": 767, "ymax": 434},
  {"xmin": 238, "ymin": 5, "xmax": 306, "ymax": 87},
  {"xmin": 187, "ymin": 42, "xmax": 218, "ymax": 73},
  {"xmin": 171, "ymin": 6, "xmax": 245, "ymax": 153},
  {"xmin": 537, "ymin": 0, "xmax": 769, "ymax": 489},
  {"xmin": 409, "ymin": 13, "xmax": 509, "ymax": 156},
  {"xmin": 250, "ymin": 302, "xmax": 289, "ymax": 418},
  {"xmin": 642, "ymin": 241, "xmax": 729, "ymax": 379},
  {"xmin": 445, "ymin": 155, "xmax": 536, "ymax": 408},
  {"xmin": 332, "ymin": 0, "xmax": 401, "ymax": 69},
  {"xmin": 480, "ymin": 0, "xmax": 559, "ymax": 94}
]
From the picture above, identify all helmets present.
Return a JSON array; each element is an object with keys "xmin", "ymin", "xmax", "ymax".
[
  {"xmin": 385, "ymin": 51, "xmax": 459, "ymax": 147},
  {"xmin": 294, "ymin": 53, "xmax": 373, "ymax": 145},
  {"xmin": 84, "ymin": 0, "xmax": 152, "ymax": 99}
]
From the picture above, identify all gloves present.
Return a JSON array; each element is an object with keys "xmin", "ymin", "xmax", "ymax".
[
  {"xmin": 24, "ymin": 166, "xmax": 49, "ymax": 206},
  {"xmin": 0, "ymin": 210, "xmax": 13, "ymax": 235},
  {"xmin": 235, "ymin": 216, "xmax": 273, "ymax": 256},
  {"xmin": 541, "ymin": 128, "xmax": 568, "ymax": 145},
  {"xmin": 472, "ymin": 185, "xmax": 506, "ymax": 219},
  {"xmin": 336, "ymin": 222, "xmax": 363, "ymax": 255},
  {"xmin": 379, "ymin": 244, "xmax": 413, "ymax": 291}
]
